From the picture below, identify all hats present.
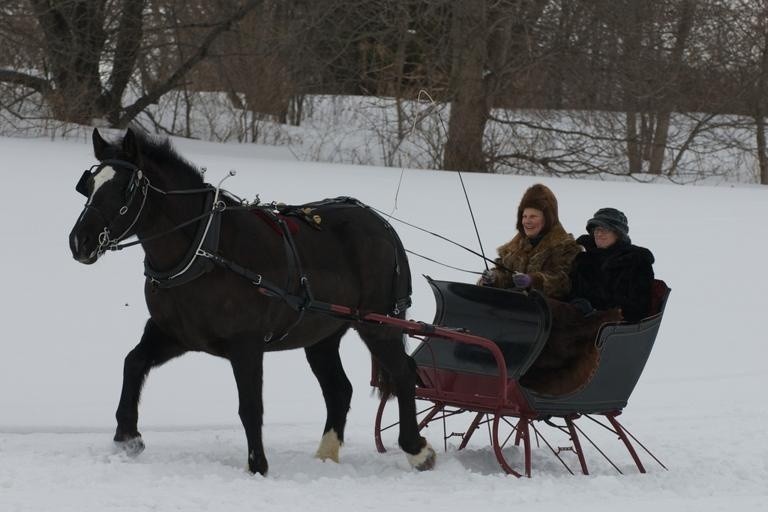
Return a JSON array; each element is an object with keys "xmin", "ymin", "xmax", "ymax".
[
  {"xmin": 586, "ymin": 208, "xmax": 629, "ymax": 239},
  {"xmin": 516, "ymin": 184, "xmax": 558, "ymax": 237}
]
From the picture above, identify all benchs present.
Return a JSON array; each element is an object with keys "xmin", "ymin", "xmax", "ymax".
[{"xmin": 426, "ymin": 276, "xmax": 665, "ymax": 413}]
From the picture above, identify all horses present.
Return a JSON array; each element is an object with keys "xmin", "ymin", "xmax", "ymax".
[{"xmin": 67, "ymin": 125, "xmax": 437, "ymax": 475}]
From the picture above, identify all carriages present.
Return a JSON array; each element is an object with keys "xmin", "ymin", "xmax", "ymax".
[{"xmin": 68, "ymin": 125, "xmax": 672, "ymax": 479}]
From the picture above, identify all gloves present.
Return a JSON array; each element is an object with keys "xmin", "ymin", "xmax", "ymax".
[
  {"xmin": 511, "ymin": 271, "xmax": 532, "ymax": 290},
  {"xmin": 573, "ymin": 297, "xmax": 604, "ymax": 325},
  {"xmin": 481, "ymin": 269, "xmax": 493, "ymax": 284}
]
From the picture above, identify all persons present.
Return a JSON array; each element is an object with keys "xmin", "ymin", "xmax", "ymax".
[
  {"xmin": 478, "ymin": 185, "xmax": 582, "ymax": 300},
  {"xmin": 571, "ymin": 208, "xmax": 653, "ymax": 323}
]
